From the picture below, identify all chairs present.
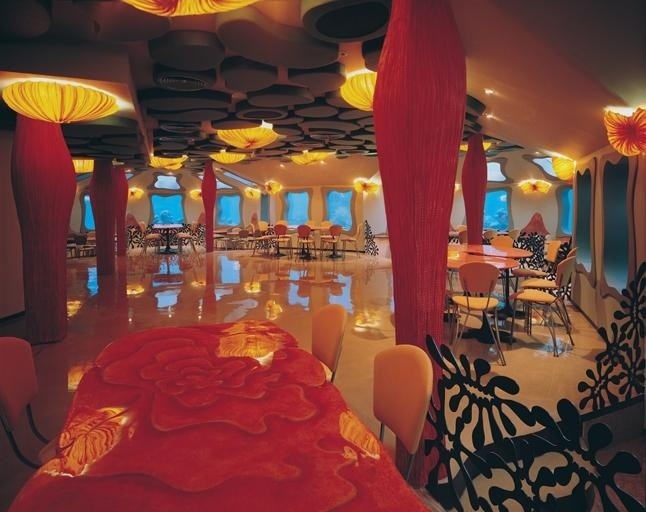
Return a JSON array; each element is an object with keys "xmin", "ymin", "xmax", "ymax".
[
  {"xmin": 444, "ymin": 210, "xmax": 581, "ymax": 366},
  {"xmin": 64, "ymin": 212, "xmax": 364, "ymax": 261},
  {"xmin": 310, "ymin": 303, "xmax": 348, "ymax": 384},
  {"xmin": 369, "ymin": 344, "xmax": 432, "ymax": 480},
  {"xmin": 0, "ymin": 337, "xmax": 51, "ymax": 470}
]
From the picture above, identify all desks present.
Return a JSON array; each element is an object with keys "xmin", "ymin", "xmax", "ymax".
[{"xmin": 7, "ymin": 320, "xmax": 433, "ymax": 512}]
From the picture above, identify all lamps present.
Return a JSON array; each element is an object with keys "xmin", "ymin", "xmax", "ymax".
[
  {"xmin": 459, "ymin": 142, "xmax": 491, "ymax": 152},
  {"xmin": 50, "ymin": 270, "xmax": 391, "ymax": 340},
  {"xmin": 0, "ymin": 76, "xmax": 123, "ymax": 125},
  {"xmin": 120, "ymin": 0, "xmax": 257, "ymax": 19},
  {"xmin": 338, "ymin": 66, "xmax": 377, "ymax": 112},
  {"xmin": 1, "ymin": 150, "xmax": 578, "ymax": 201},
  {"xmin": 216, "ymin": 120, "xmax": 279, "ymax": 150},
  {"xmin": 602, "ymin": 103, "xmax": 646, "ymax": 158}
]
